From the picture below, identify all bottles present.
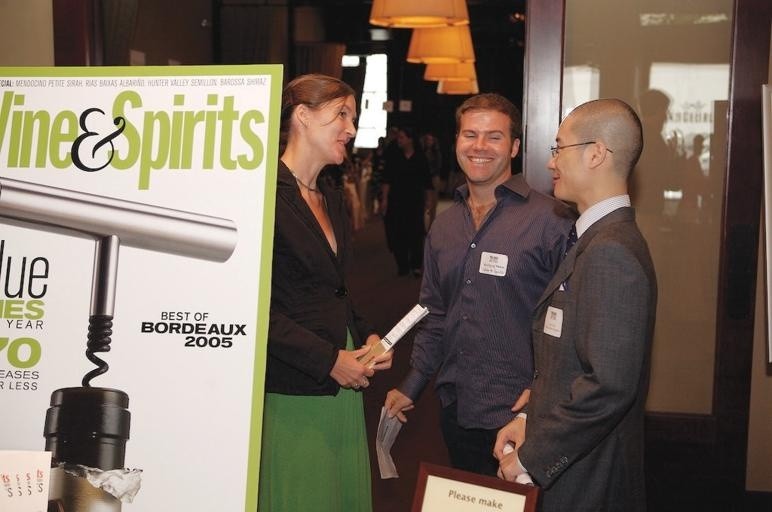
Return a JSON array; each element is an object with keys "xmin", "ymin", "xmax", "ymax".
[{"xmin": 39, "ymin": 388, "xmax": 133, "ymax": 512}]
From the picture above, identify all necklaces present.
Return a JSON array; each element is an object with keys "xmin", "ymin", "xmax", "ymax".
[{"xmin": 291, "ymin": 171, "xmax": 318, "ymax": 193}]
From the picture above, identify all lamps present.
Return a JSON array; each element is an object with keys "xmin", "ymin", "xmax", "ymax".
[
  {"xmin": 365, "ymin": 1, "xmax": 470, "ymax": 28},
  {"xmin": 436, "ymin": 81, "xmax": 479, "ymax": 96},
  {"xmin": 422, "ymin": 64, "xmax": 477, "ymax": 81},
  {"xmin": 407, "ymin": 28, "xmax": 477, "ymax": 64}
]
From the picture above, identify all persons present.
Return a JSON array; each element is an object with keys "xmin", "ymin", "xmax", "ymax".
[
  {"xmin": 257, "ymin": 73, "xmax": 395, "ymax": 512},
  {"xmin": 363, "ymin": 120, "xmax": 447, "ymax": 279},
  {"xmin": 631, "ymin": 89, "xmax": 708, "ymax": 229},
  {"xmin": 493, "ymin": 97, "xmax": 658, "ymax": 512},
  {"xmin": 383, "ymin": 91, "xmax": 581, "ymax": 479}
]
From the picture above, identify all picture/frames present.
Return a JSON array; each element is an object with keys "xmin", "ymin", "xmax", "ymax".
[{"xmin": 410, "ymin": 461, "xmax": 540, "ymax": 512}]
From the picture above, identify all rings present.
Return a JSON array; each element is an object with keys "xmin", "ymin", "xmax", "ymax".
[
  {"xmin": 360, "ymin": 379, "xmax": 366, "ymax": 387},
  {"xmin": 352, "ymin": 383, "xmax": 359, "ymax": 389}
]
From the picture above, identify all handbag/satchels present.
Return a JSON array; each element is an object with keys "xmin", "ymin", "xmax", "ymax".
[{"xmin": 424, "ymin": 210, "xmax": 431, "ymax": 233}]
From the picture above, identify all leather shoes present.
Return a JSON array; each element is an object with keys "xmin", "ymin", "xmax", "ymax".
[{"xmin": 413, "ymin": 268, "xmax": 422, "ymax": 277}]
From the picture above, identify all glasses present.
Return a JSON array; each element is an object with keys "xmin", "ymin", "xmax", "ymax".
[{"xmin": 551, "ymin": 142, "xmax": 613, "ymax": 158}]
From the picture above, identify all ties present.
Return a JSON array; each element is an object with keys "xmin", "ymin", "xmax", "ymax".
[{"xmin": 563, "ymin": 224, "xmax": 577, "ymax": 260}]
